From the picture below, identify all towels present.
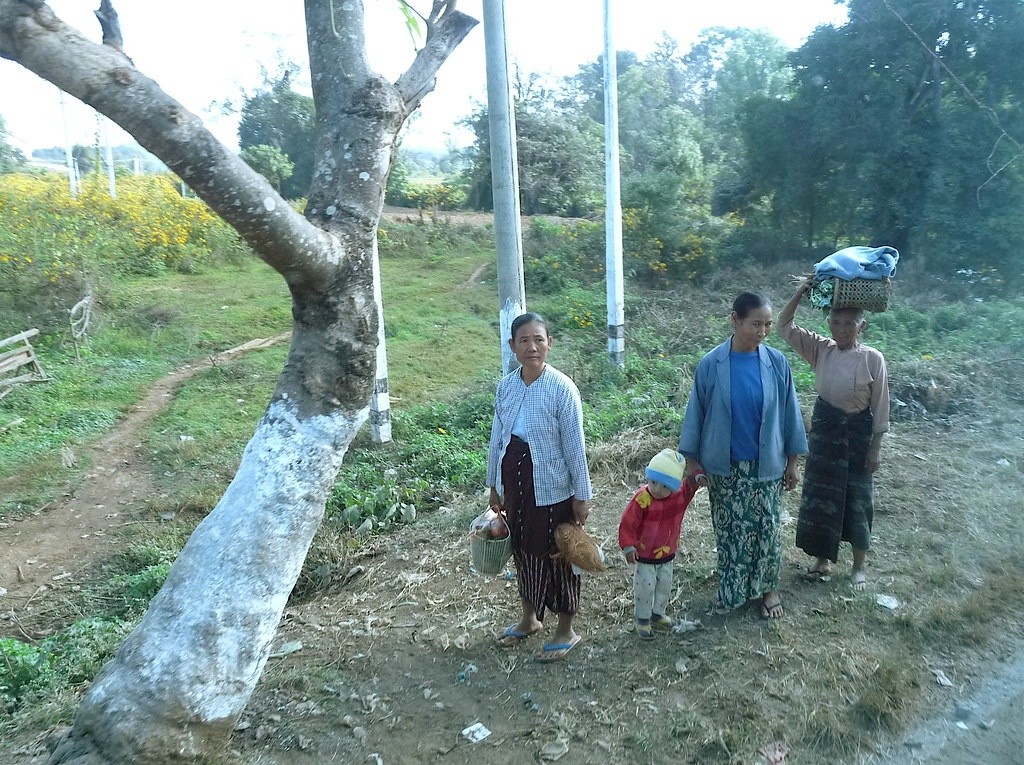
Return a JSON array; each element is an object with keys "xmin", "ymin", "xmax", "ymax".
[{"xmin": 812, "ymin": 243, "xmax": 901, "ymax": 285}]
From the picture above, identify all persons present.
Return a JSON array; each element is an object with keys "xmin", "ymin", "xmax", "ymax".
[
  {"xmin": 617, "ymin": 447, "xmax": 711, "ymax": 640},
  {"xmin": 486, "ymin": 313, "xmax": 593, "ymax": 661},
  {"xmin": 677, "ymin": 291, "xmax": 810, "ymax": 621},
  {"xmin": 774, "ymin": 277, "xmax": 891, "ymax": 590}
]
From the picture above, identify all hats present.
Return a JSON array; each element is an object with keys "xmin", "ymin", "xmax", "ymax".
[{"xmin": 645, "ymin": 448, "xmax": 685, "ymax": 492}]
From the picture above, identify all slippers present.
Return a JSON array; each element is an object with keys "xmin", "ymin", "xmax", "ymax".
[
  {"xmin": 802, "ymin": 566, "xmax": 833, "ymax": 580},
  {"xmin": 652, "ymin": 612, "xmax": 678, "ymax": 630},
  {"xmin": 760, "ymin": 598, "xmax": 783, "ymax": 621},
  {"xmin": 711, "ymin": 590, "xmax": 723, "ymax": 608},
  {"xmin": 633, "ymin": 621, "xmax": 656, "ymax": 639},
  {"xmin": 497, "ymin": 620, "xmax": 543, "ymax": 647},
  {"xmin": 534, "ymin": 634, "xmax": 583, "ymax": 663},
  {"xmin": 848, "ymin": 578, "xmax": 866, "ymax": 592}
]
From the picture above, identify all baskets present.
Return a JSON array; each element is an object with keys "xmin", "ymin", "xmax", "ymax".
[
  {"xmin": 468, "ymin": 504, "xmax": 512, "ymax": 576},
  {"xmin": 813, "ymin": 276, "xmax": 890, "ymax": 312}
]
